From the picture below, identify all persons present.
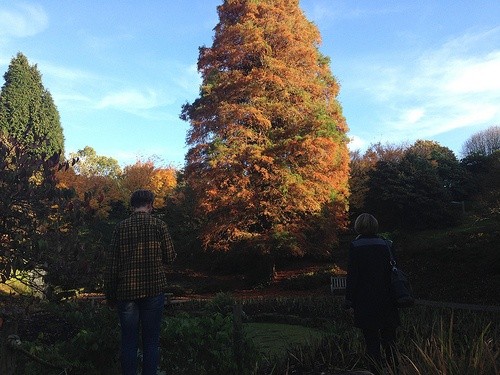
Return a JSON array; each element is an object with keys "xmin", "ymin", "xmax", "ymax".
[
  {"xmin": 103, "ymin": 189, "xmax": 178, "ymax": 375},
  {"xmin": 344, "ymin": 212, "xmax": 403, "ymax": 374}
]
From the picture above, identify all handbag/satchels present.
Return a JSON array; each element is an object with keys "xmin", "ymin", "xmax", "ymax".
[{"xmin": 381, "ymin": 236, "xmax": 414, "ymax": 309}]
